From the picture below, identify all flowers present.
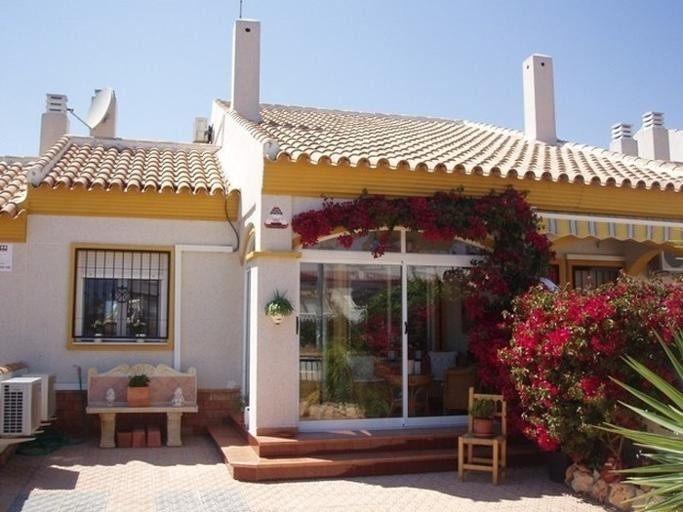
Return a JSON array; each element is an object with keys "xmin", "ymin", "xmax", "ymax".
[
  {"xmin": 495, "ymin": 268, "xmax": 683, "ymax": 461},
  {"xmin": 292, "ymin": 179, "xmax": 559, "ymax": 294}
]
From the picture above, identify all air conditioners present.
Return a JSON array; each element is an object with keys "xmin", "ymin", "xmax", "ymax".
[
  {"xmin": 649, "ymin": 249, "xmax": 683, "ymax": 275},
  {"xmin": 0, "ymin": 371, "xmax": 56, "ymax": 438}
]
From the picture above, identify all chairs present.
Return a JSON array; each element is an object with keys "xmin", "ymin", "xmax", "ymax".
[
  {"xmin": 456, "ymin": 386, "xmax": 508, "ymax": 486},
  {"xmin": 374, "ymin": 348, "xmax": 486, "ymax": 412}
]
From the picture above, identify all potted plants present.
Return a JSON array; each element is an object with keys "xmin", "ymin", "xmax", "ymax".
[
  {"xmin": 264, "ymin": 286, "xmax": 295, "ymax": 323},
  {"xmin": 127, "ymin": 374, "xmax": 151, "ymax": 405},
  {"xmin": 471, "ymin": 399, "xmax": 497, "ymax": 438}
]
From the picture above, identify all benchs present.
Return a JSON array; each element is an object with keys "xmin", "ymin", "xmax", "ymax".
[{"xmin": 85, "ymin": 362, "xmax": 198, "ymax": 449}]
